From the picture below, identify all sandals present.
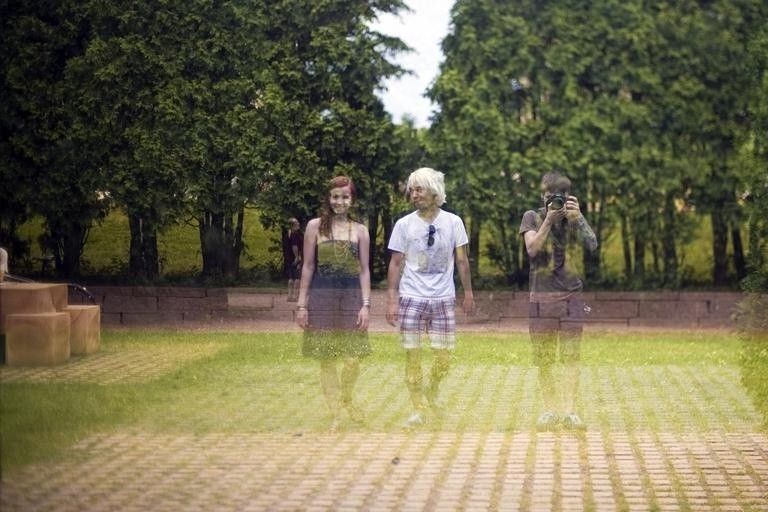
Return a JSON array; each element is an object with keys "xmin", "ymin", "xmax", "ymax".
[
  {"xmin": 323, "ymin": 413, "xmax": 342, "ymax": 438},
  {"xmin": 343, "ymin": 396, "xmax": 367, "ymax": 425}
]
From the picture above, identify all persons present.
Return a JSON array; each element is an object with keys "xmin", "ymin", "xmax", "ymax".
[
  {"xmin": 518, "ymin": 169, "xmax": 600, "ymax": 429},
  {"xmin": 282, "ymin": 217, "xmax": 299, "ymax": 302},
  {"xmin": 384, "ymin": 164, "xmax": 477, "ymax": 429},
  {"xmin": 293, "ymin": 175, "xmax": 374, "ymax": 436},
  {"xmin": 284, "ymin": 221, "xmax": 308, "ymax": 267}
]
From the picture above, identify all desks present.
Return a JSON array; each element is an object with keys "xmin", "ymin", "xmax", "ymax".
[{"xmin": 0, "ymin": 281, "xmax": 68, "ymax": 341}]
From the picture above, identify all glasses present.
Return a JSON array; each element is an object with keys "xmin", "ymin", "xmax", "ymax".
[{"xmin": 428, "ymin": 223, "xmax": 435, "ymax": 247}]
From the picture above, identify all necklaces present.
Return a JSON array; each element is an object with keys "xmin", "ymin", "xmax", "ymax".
[{"xmin": 329, "ymin": 218, "xmax": 353, "ymax": 264}]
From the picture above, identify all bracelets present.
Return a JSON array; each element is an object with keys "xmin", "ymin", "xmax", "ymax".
[
  {"xmin": 295, "ymin": 305, "xmax": 305, "ymax": 312},
  {"xmin": 360, "ymin": 296, "xmax": 370, "ymax": 307}
]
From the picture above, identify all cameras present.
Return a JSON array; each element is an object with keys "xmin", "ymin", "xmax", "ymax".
[{"xmin": 546, "ymin": 194, "xmax": 566, "ymax": 212}]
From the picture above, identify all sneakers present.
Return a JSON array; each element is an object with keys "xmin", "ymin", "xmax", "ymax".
[
  {"xmin": 422, "ymin": 384, "xmax": 447, "ymax": 420},
  {"xmin": 534, "ymin": 408, "xmax": 561, "ymax": 432},
  {"xmin": 562, "ymin": 413, "xmax": 588, "ymax": 435},
  {"xmin": 399, "ymin": 410, "xmax": 427, "ymax": 432}
]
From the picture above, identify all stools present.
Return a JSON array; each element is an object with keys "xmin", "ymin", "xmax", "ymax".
[
  {"xmin": 66, "ymin": 305, "xmax": 100, "ymax": 354},
  {"xmin": 6, "ymin": 312, "xmax": 70, "ymax": 366}
]
